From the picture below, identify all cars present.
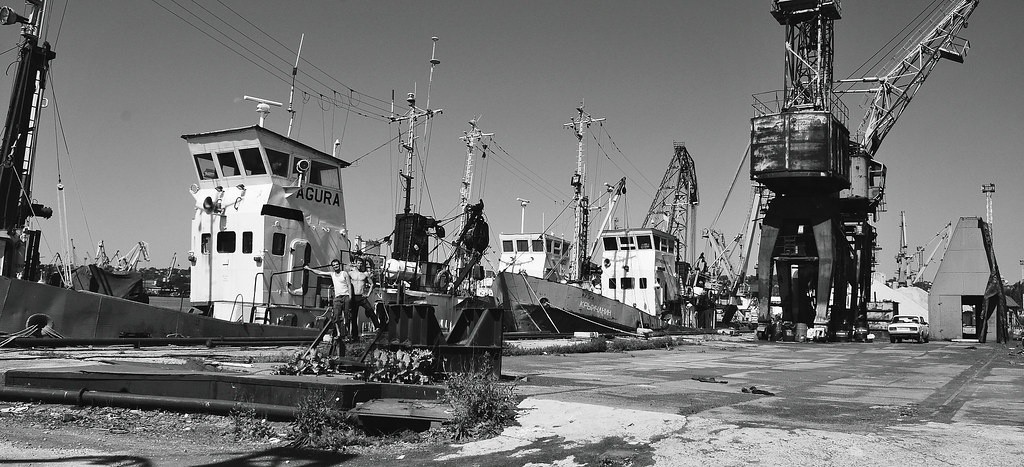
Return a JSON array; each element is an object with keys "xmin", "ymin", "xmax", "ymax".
[{"xmin": 887, "ymin": 315, "xmax": 929, "ymax": 345}]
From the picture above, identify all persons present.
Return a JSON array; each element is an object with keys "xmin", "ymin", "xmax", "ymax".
[
  {"xmin": 304, "ymin": 259, "xmax": 353, "ymax": 341},
  {"xmin": 347, "ymin": 258, "xmax": 380, "ymax": 343}
]
